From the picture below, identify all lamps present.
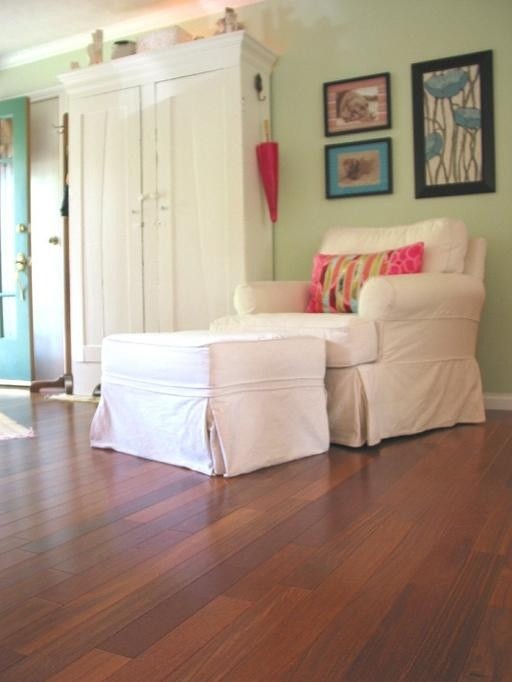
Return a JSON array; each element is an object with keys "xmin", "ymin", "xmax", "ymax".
[{"xmin": 234, "ymin": 219, "xmax": 487, "ymax": 450}]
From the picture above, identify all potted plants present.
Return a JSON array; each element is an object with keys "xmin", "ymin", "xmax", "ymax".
[
  {"xmin": 410, "ymin": 49, "xmax": 497, "ymax": 199},
  {"xmin": 322, "ymin": 71, "xmax": 394, "ymax": 199}
]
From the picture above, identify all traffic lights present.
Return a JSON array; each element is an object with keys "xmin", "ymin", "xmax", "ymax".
[{"xmin": 304, "ymin": 219, "xmax": 467, "ymax": 314}]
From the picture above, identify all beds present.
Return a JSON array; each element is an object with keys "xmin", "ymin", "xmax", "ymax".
[{"xmin": 253, "ymin": 118, "xmax": 283, "ymax": 223}]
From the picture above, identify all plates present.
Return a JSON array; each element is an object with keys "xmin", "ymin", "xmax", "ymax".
[{"xmin": 59, "ymin": 30, "xmax": 278, "ymax": 397}]
